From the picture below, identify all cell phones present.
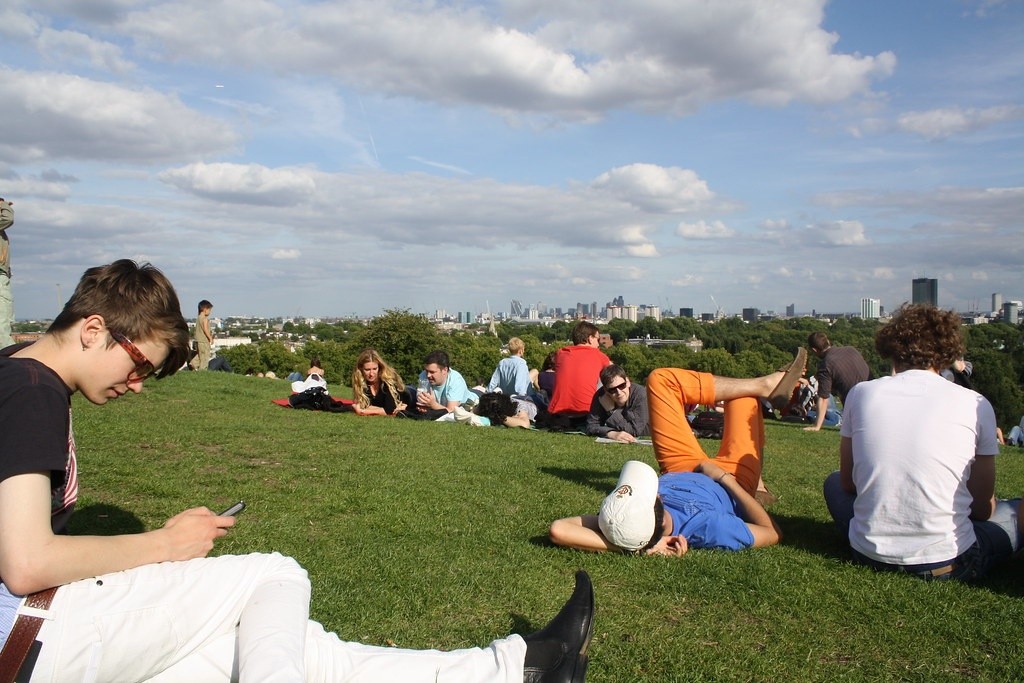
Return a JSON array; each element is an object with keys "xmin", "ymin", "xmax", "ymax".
[{"xmin": 219, "ymin": 501, "xmax": 246, "ymax": 516}]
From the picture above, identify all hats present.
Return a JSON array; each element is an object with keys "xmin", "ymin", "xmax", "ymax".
[{"xmin": 598, "ymin": 460, "xmax": 658, "ymax": 552}]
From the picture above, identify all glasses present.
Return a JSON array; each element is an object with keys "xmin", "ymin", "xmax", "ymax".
[
  {"xmin": 603, "ymin": 378, "xmax": 626, "ymax": 394},
  {"xmin": 107, "ymin": 326, "xmax": 155, "ymax": 382}
]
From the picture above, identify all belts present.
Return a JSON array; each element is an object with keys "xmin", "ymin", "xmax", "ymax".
[{"xmin": 0, "ymin": 586, "xmax": 59, "ymax": 683}]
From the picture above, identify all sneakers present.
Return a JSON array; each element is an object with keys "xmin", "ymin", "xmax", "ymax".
[
  {"xmin": 766, "ymin": 347, "xmax": 808, "ymax": 410},
  {"xmin": 754, "ymin": 485, "xmax": 775, "ymax": 505}
]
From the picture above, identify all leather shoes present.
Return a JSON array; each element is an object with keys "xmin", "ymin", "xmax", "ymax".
[{"xmin": 521, "ymin": 571, "xmax": 594, "ymax": 683}]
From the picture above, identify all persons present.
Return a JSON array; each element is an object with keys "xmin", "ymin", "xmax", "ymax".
[
  {"xmin": 0, "ymin": 342, "xmax": 77, "ymax": 533},
  {"xmin": 997, "ymin": 416, "xmax": 1024, "ymax": 447},
  {"xmin": 306, "ymin": 358, "xmax": 324, "ymax": 378},
  {"xmin": 416, "ymin": 350, "xmax": 486, "ymax": 414},
  {"xmin": 189, "ymin": 300, "xmax": 214, "ymax": 371},
  {"xmin": 761, "ymin": 332, "xmax": 875, "ymax": 432},
  {"xmin": 187, "ymin": 341, "xmax": 234, "ymax": 371},
  {"xmin": 549, "ymin": 347, "xmax": 808, "ymax": 559},
  {"xmin": 0, "ymin": 198, "xmax": 17, "ymax": 351},
  {"xmin": 0, "ymin": 259, "xmax": 594, "ymax": 683},
  {"xmin": 823, "ymin": 301, "xmax": 1024, "ymax": 598},
  {"xmin": 352, "ymin": 348, "xmax": 418, "ymax": 417},
  {"xmin": 242, "ymin": 367, "xmax": 304, "ymax": 382},
  {"xmin": 473, "ymin": 320, "xmax": 724, "ymax": 445},
  {"xmin": 939, "ymin": 355, "xmax": 982, "ymax": 394}
]
